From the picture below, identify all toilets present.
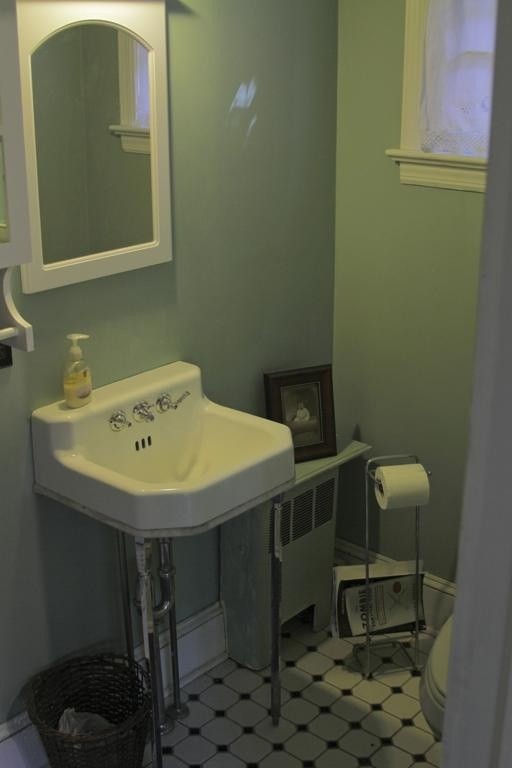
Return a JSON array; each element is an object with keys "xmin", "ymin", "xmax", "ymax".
[{"xmin": 419, "ymin": 611, "xmax": 454, "ymax": 738}]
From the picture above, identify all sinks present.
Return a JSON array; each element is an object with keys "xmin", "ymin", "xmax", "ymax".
[{"xmin": 30, "ymin": 361, "xmax": 295, "ymax": 530}]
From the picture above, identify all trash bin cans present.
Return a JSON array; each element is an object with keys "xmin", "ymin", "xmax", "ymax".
[{"xmin": 22, "ymin": 653, "xmax": 153, "ymax": 768}]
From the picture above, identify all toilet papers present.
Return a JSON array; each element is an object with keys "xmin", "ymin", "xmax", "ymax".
[{"xmin": 374, "ymin": 464, "xmax": 430, "ymax": 510}]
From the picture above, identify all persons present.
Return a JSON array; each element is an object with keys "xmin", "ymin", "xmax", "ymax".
[{"xmin": 293, "ymin": 400, "xmax": 308, "ymax": 418}]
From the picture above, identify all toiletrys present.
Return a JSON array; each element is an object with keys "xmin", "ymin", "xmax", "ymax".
[{"xmin": 63, "ymin": 333, "xmax": 91, "ymax": 409}]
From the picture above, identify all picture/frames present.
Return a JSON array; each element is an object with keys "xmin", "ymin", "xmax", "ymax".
[{"xmin": 263, "ymin": 364, "xmax": 337, "ymax": 464}]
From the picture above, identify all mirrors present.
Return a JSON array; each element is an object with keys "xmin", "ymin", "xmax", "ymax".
[{"xmin": 10, "ymin": 1, "xmax": 174, "ymax": 294}]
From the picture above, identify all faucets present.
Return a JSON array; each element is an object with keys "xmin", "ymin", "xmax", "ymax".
[{"xmin": 133, "ymin": 403, "xmax": 155, "ymax": 422}]
